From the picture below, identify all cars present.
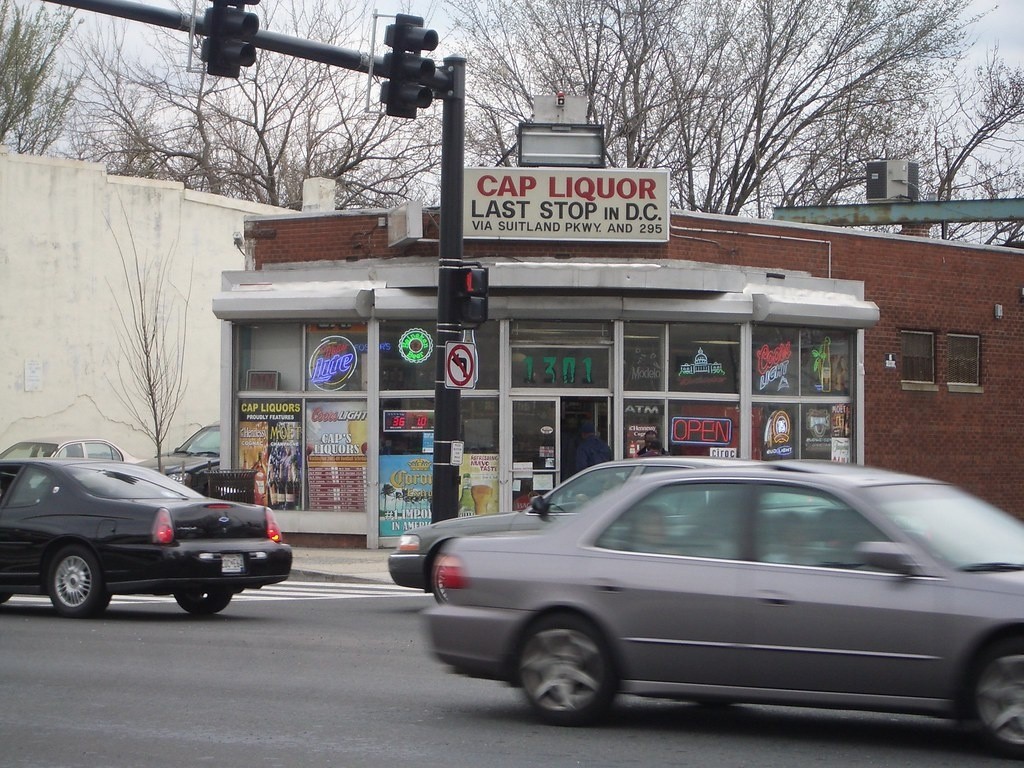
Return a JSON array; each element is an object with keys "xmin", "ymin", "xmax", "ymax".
[
  {"xmin": 418, "ymin": 461, "xmax": 1024, "ymax": 763},
  {"xmin": 0, "ymin": 434, "xmax": 149, "ymax": 489},
  {"xmin": 0, "ymin": 458, "xmax": 294, "ymax": 619},
  {"xmin": 136, "ymin": 421, "xmax": 226, "ymax": 498},
  {"xmin": 386, "ymin": 456, "xmax": 845, "ymax": 605}
]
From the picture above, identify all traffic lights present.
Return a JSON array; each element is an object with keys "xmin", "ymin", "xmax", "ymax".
[
  {"xmin": 448, "ymin": 267, "xmax": 490, "ymax": 325},
  {"xmin": 379, "ymin": 13, "xmax": 440, "ymax": 119},
  {"xmin": 202, "ymin": 0, "xmax": 261, "ymax": 81}
]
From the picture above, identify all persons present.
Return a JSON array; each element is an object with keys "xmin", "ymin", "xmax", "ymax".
[
  {"xmin": 759, "ymin": 512, "xmax": 845, "ymax": 565},
  {"xmin": 640, "ymin": 440, "xmax": 674, "ymax": 456},
  {"xmin": 637, "ymin": 431, "xmax": 656, "ymax": 455},
  {"xmin": 574, "ymin": 422, "xmax": 612, "ymax": 471},
  {"xmin": 630, "ymin": 503, "xmax": 672, "ymax": 548}
]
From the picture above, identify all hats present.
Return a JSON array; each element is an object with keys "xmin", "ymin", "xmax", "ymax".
[{"xmin": 576, "ymin": 423, "xmax": 595, "ymax": 434}]
